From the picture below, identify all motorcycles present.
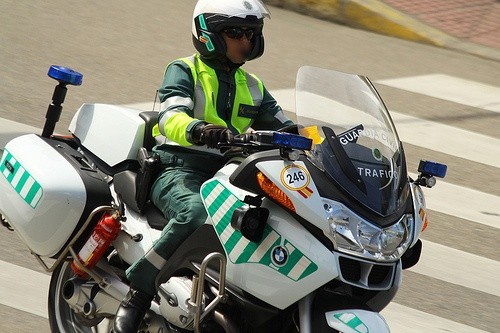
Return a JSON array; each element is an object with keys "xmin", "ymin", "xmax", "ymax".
[{"xmin": 1, "ymin": 64, "xmax": 447, "ymax": 333}]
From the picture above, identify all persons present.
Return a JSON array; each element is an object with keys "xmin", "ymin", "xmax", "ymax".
[{"xmin": 113, "ymin": 0, "xmax": 298, "ymax": 333}]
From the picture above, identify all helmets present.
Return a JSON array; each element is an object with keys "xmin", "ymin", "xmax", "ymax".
[{"xmin": 191, "ymin": 0, "xmax": 271, "ymax": 67}]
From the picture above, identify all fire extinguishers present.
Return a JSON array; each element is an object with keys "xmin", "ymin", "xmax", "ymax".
[{"xmin": 70, "ymin": 199, "xmax": 127, "ymax": 278}]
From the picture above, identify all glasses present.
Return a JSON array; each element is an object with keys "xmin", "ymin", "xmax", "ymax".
[{"xmin": 222, "ymin": 26, "xmax": 255, "ymax": 41}]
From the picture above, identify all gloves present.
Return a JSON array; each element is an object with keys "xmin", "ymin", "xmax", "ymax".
[{"xmin": 194, "ymin": 124, "xmax": 230, "ymax": 149}]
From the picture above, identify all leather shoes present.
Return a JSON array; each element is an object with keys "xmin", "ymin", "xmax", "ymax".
[{"xmin": 112, "ymin": 286, "xmax": 155, "ymax": 332}]
